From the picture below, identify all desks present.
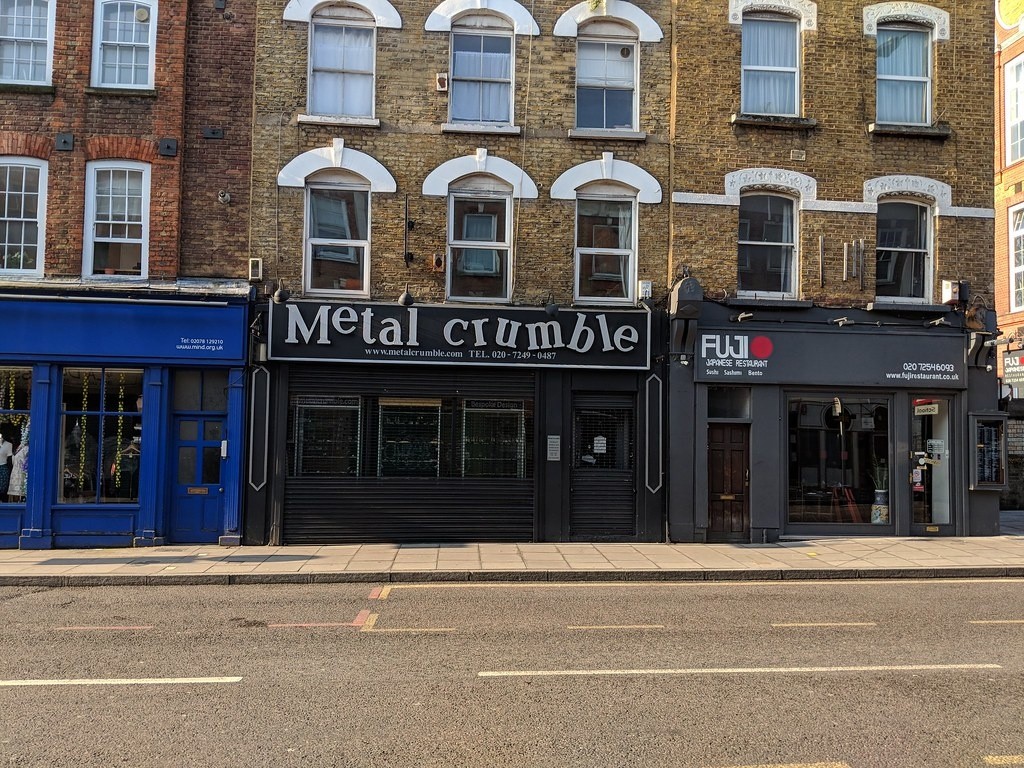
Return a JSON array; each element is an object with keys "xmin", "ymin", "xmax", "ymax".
[{"xmin": 803, "ymin": 493, "xmax": 833, "ymax": 522}]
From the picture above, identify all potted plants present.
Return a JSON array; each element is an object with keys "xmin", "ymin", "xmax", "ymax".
[{"xmin": 867, "ymin": 449, "xmax": 888, "ymax": 523}]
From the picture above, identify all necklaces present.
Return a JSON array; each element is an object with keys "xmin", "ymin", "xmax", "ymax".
[{"xmin": 13, "ymin": 445, "xmax": 24, "ymax": 455}]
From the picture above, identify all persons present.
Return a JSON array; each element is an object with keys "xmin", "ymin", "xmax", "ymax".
[
  {"xmin": 6, "ymin": 438, "xmax": 29, "ymax": 502},
  {"xmin": 0, "ymin": 433, "xmax": 13, "ymax": 503}
]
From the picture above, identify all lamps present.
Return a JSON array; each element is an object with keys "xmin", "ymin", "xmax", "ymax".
[
  {"xmin": 833, "ymin": 317, "xmax": 855, "ymax": 327},
  {"xmin": 542, "ymin": 293, "xmax": 558, "ymax": 316},
  {"xmin": 929, "ymin": 316, "xmax": 951, "ymax": 327},
  {"xmin": 737, "ymin": 312, "xmax": 754, "ymax": 322},
  {"xmin": 274, "ymin": 278, "xmax": 291, "ymax": 304},
  {"xmin": 398, "ymin": 283, "xmax": 414, "ymax": 306}
]
